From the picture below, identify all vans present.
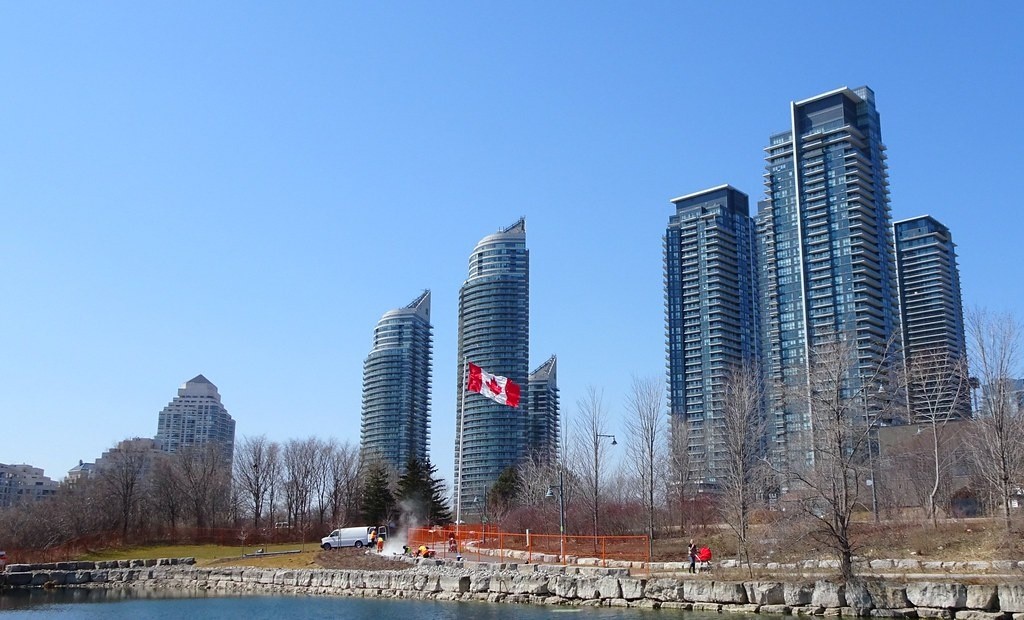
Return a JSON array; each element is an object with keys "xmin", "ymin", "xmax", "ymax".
[{"xmin": 320, "ymin": 525, "xmax": 387, "ymax": 552}]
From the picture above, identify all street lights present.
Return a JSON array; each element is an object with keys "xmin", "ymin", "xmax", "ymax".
[
  {"xmin": 473, "ymin": 485, "xmax": 486, "ymax": 545},
  {"xmin": 593, "ymin": 434, "xmax": 618, "ymax": 552},
  {"xmin": 546, "ymin": 471, "xmax": 567, "ymax": 565},
  {"xmin": 862, "ymin": 374, "xmax": 887, "ymax": 525}
]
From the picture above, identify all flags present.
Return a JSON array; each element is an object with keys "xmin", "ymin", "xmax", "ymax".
[{"xmin": 467, "ymin": 361, "xmax": 520, "ymax": 408}]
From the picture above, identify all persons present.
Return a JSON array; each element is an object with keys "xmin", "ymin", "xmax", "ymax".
[
  {"xmin": 416, "ymin": 544, "xmax": 430, "ymax": 557},
  {"xmin": 403, "ymin": 545, "xmax": 412, "ymax": 556},
  {"xmin": 449, "ymin": 531, "xmax": 455, "ymax": 544},
  {"xmin": 377, "ymin": 537, "xmax": 384, "ymax": 553},
  {"xmin": 688, "ymin": 539, "xmax": 698, "ymax": 574},
  {"xmin": 370, "ymin": 528, "xmax": 378, "ymax": 547}
]
